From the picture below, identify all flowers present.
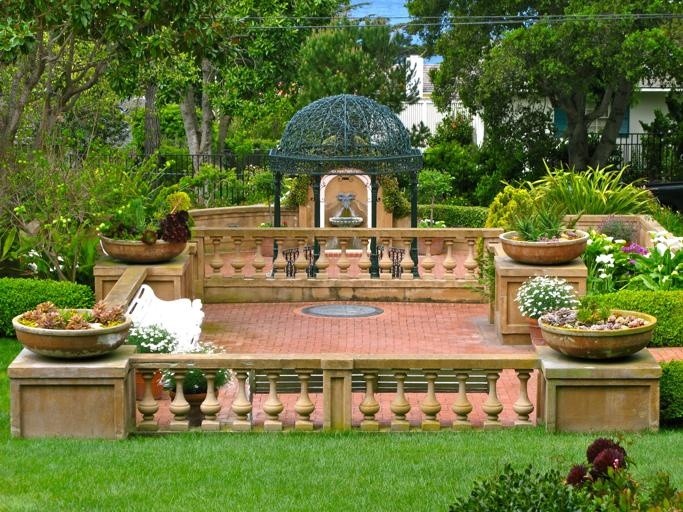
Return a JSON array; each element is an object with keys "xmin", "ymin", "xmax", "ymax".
[
  {"xmin": 93, "ymin": 189, "xmax": 191, "ymax": 244},
  {"xmin": 541, "ymin": 292, "xmax": 648, "ymax": 332},
  {"xmin": 513, "ymin": 273, "xmax": 580, "ymax": 321},
  {"xmin": 505, "ymin": 195, "xmax": 587, "ymax": 243},
  {"xmin": 416, "ymin": 217, "xmax": 445, "ymax": 228},
  {"xmin": 257, "ymin": 220, "xmax": 289, "ymax": 228},
  {"xmin": 16, "ymin": 295, "xmax": 124, "ymax": 330},
  {"xmin": 159, "ymin": 341, "xmax": 233, "ymax": 394},
  {"xmin": 123, "ymin": 321, "xmax": 178, "ymax": 354}
]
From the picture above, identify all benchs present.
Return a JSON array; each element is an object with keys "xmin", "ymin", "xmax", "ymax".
[{"xmin": 124, "ymin": 279, "xmax": 204, "ymax": 351}]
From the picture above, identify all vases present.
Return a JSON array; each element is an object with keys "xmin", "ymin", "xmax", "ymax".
[
  {"xmin": 526, "ymin": 317, "xmax": 546, "ymax": 351},
  {"xmin": 416, "ymin": 236, "xmax": 443, "ymax": 255},
  {"xmin": 9, "ymin": 307, "xmax": 135, "ymax": 361},
  {"xmin": 95, "ymin": 229, "xmax": 188, "ymax": 264},
  {"xmin": 135, "ymin": 369, "xmax": 164, "ymax": 400},
  {"xmin": 536, "ymin": 307, "xmax": 659, "ymax": 362},
  {"xmin": 498, "ymin": 227, "xmax": 591, "ymax": 266},
  {"xmin": 259, "ymin": 237, "xmax": 286, "ymax": 256},
  {"xmin": 168, "ymin": 390, "xmax": 218, "ymax": 412}
]
